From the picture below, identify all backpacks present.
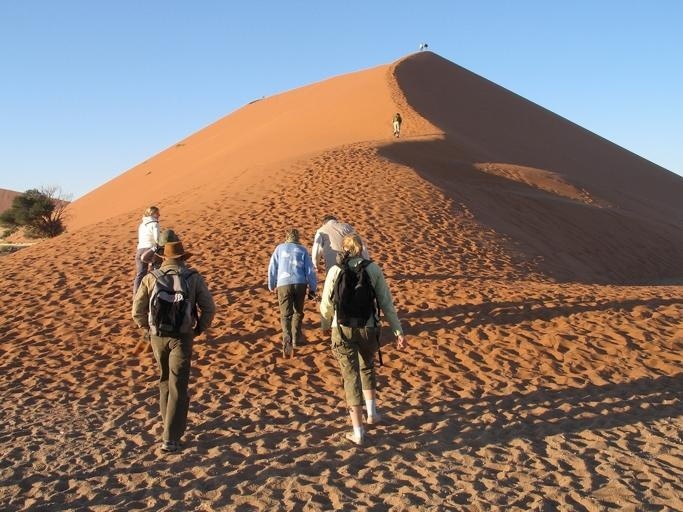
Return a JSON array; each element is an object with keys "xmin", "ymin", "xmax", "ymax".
[{"xmin": 149, "ymin": 266, "xmax": 199, "ymax": 338}]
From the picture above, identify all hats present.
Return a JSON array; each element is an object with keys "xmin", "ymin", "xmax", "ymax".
[{"xmin": 153, "ymin": 241, "xmax": 193, "ymax": 263}]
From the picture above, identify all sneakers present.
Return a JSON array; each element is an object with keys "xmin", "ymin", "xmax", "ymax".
[
  {"xmin": 160, "ymin": 439, "xmax": 183, "ymax": 451},
  {"xmin": 365, "ymin": 413, "xmax": 382, "ymax": 426},
  {"xmin": 345, "ymin": 430, "xmax": 365, "ymax": 446},
  {"xmin": 280, "ymin": 333, "xmax": 306, "ymax": 358},
  {"xmin": 330, "ymin": 259, "xmax": 375, "ymax": 328}
]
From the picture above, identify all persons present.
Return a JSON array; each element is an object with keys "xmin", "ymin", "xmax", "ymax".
[
  {"xmin": 419, "ymin": 41, "xmax": 423, "ymax": 52},
  {"xmin": 130, "ymin": 206, "xmax": 163, "ymax": 305},
  {"xmin": 139, "ymin": 229, "xmax": 180, "ymax": 270},
  {"xmin": 310, "ymin": 215, "xmax": 370, "ymax": 276},
  {"xmin": 130, "ymin": 241, "xmax": 216, "ymax": 452},
  {"xmin": 267, "ymin": 227, "xmax": 317, "ymax": 360},
  {"xmin": 318, "ymin": 232, "xmax": 407, "ymax": 446},
  {"xmin": 392, "ymin": 112, "xmax": 402, "ymax": 138},
  {"xmin": 423, "ymin": 41, "xmax": 428, "ymax": 51}
]
